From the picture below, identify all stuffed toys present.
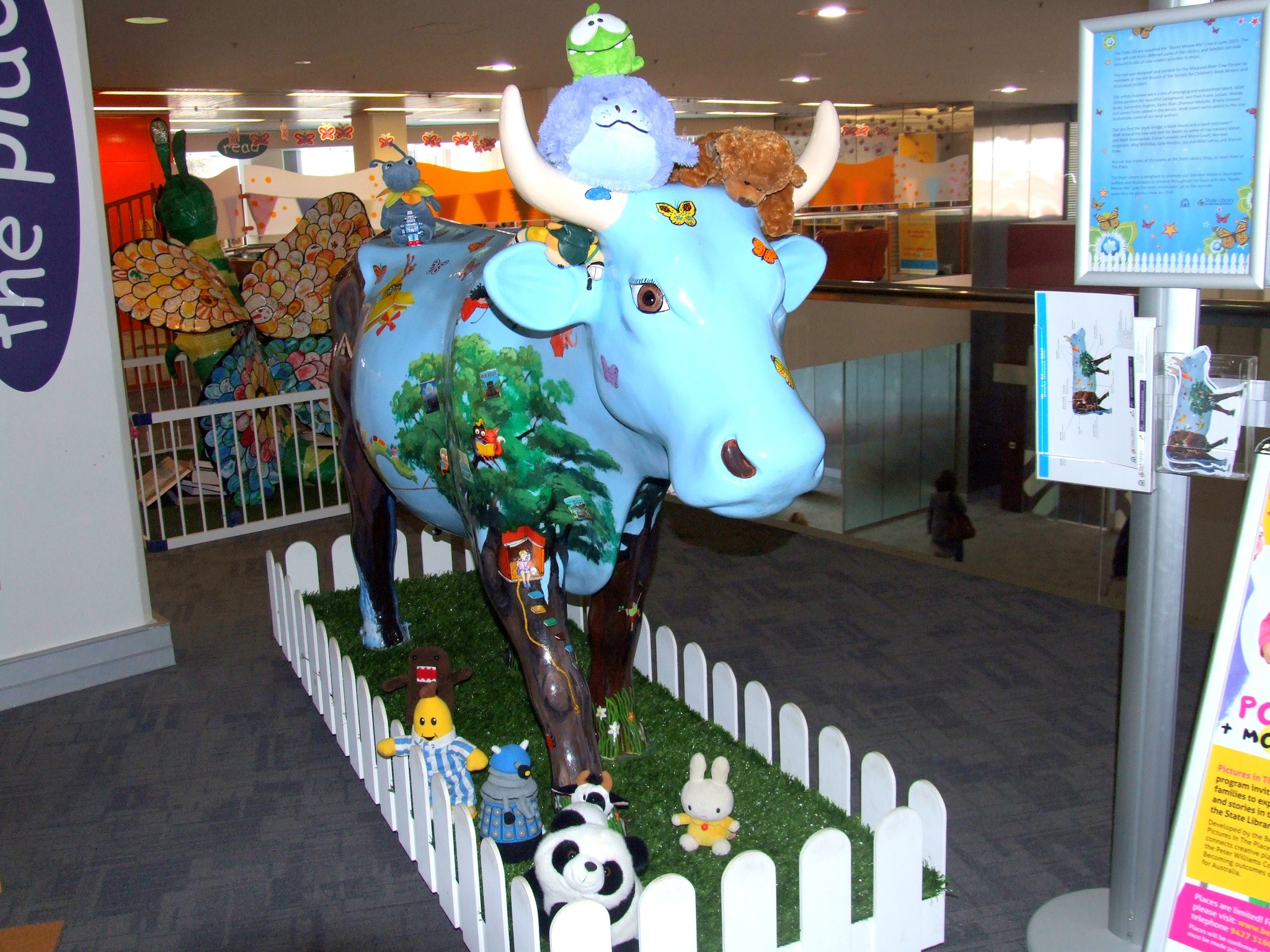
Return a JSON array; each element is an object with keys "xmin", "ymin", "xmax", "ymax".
[
  {"xmin": 369, "ymin": 142, "xmax": 443, "ymax": 246},
  {"xmin": 668, "ymin": 125, "xmax": 807, "ymax": 238},
  {"xmin": 565, "ymin": 1, "xmax": 645, "ymax": 85},
  {"xmin": 551, "ymin": 770, "xmax": 630, "ymax": 816},
  {"xmin": 375, "ymin": 695, "xmax": 489, "ymax": 825},
  {"xmin": 381, "ymin": 646, "xmax": 474, "ymax": 727},
  {"xmin": 477, "ymin": 738, "xmax": 547, "ymax": 864},
  {"xmin": 535, "ymin": 74, "xmax": 700, "ymax": 193},
  {"xmin": 671, "ymin": 752, "xmax": 740, "ymax": 856},
  {"xmin": 522, "ymin": 809, "xmax": 649, "ymax": 952}
]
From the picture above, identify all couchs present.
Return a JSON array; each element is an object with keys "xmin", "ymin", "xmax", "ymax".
[{"xmin": 812, "ymin": 226, "xmax": 888, "ymax": 282}]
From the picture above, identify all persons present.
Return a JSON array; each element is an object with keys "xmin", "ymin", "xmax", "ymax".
[
  {"xmin": 1110, "ymin": 514, "xmax": 1130, "ymax": 599},
  {"xmin": 924, "ymin": 469, "xmax": 968, "ymax": 563}
]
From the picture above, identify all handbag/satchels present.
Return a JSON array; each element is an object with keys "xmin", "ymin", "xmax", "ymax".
[{"xmin": 944, "ymin": 493, "xmax": 976, "ymax": 542}]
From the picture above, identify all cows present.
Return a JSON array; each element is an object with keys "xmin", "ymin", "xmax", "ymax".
[{"xmin": 326, "ymin": 85, "xmax": 842, "ymax": 814}]
